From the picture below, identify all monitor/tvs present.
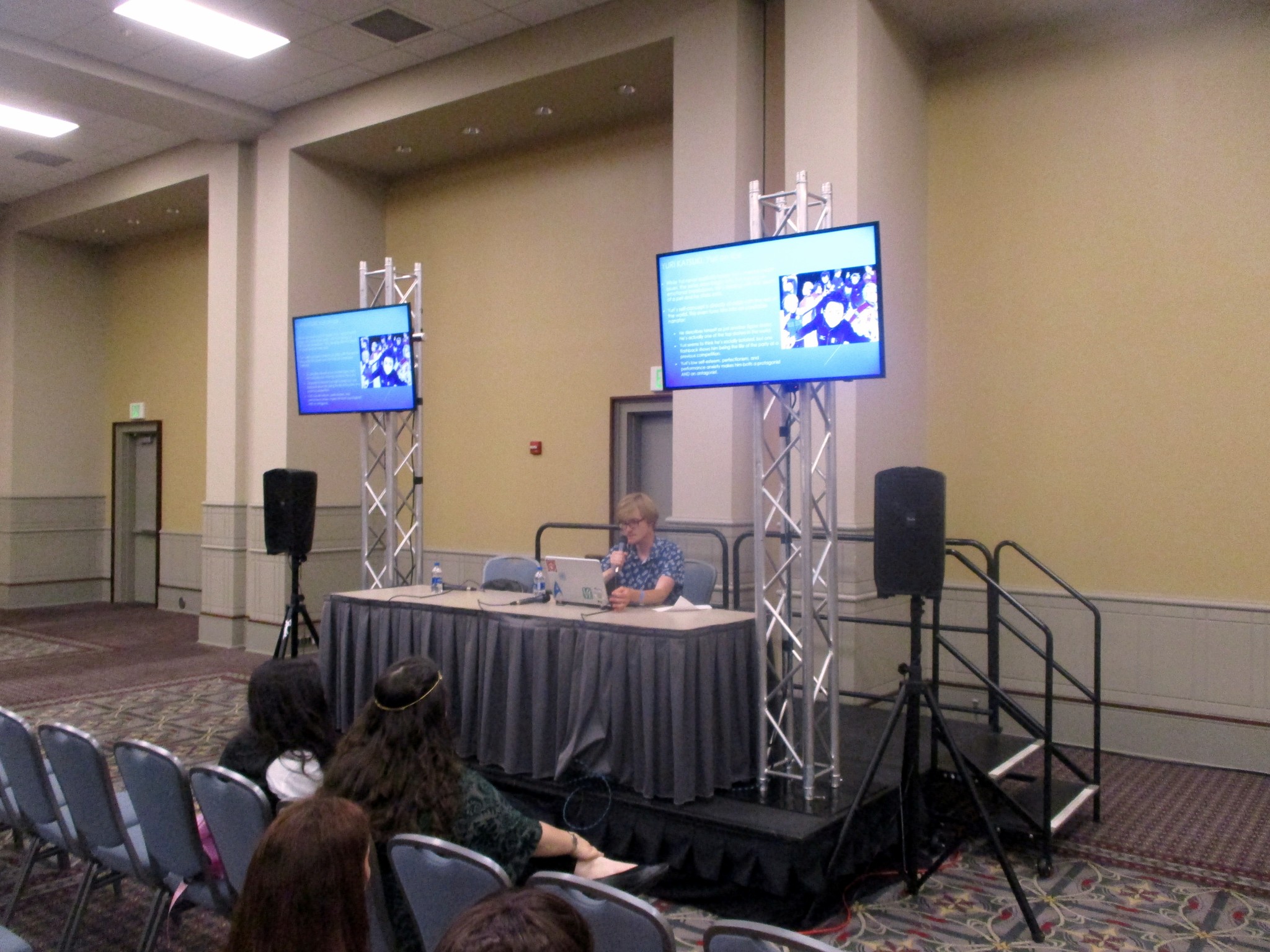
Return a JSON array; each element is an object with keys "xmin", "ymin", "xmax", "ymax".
[
  {"xmin": 655, "ymin": 219, "xmax": 886, "ymax": 394},
  {"xmin": 292, "ymin": 304, "xmax": 419, "ymax": 417}
]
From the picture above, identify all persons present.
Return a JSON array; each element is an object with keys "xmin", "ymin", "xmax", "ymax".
[
  {"xmin": 777, "ymin": 265, "xmax": 878, "ymax": 350},
  {"xmin": 316, "ymin": 656, "xmax": 668, "ymax": 897},
  {"xmin": 359, "ymin": 332, "xmax": 411, "ymax": 389},
  {"xmin": 227, "ymin": 794, "xmax": 373, "ymax": 952},
  {"xmin": 599, "ymin": 492, "xmax": 685, "ymax": 613},
  {"xmin": 203, "ymin": 658, "xmax": 340, "ymax": 897},
  {"xmin": 439, "ymin": 887, "xmax": 595, "ymax": 952}
]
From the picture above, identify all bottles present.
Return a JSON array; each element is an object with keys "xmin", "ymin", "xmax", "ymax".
[
  {"xmin": 533, "ymin": 567, "xmax": 545, "ymax": 597},
  {"xmin": 431, "ymin": 562, "xmax": 443, "ymax": 595}
]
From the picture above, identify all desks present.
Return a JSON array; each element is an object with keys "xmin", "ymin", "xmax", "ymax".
[{"xmin": 319, "ymin": 583, "xmax": 757, "ymax": 804}]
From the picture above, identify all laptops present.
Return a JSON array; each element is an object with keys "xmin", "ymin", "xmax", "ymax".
[{"xmin": 542, "ymin": 556, "xmax": 609, "ymax": 607}]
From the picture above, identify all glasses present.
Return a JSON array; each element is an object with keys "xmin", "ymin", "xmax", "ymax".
[{"xmin": 617, "ymin": 518, "xmax": 644, "ymax": 530}]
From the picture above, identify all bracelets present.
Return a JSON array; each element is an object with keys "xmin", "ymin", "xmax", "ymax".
[
  {"xmin": 568, "ymin": 830, "xmax": 578, "ymax": 853},
  {"xmin": 639, "ymin": 589, "xmax": 645, "ymax": 604}
]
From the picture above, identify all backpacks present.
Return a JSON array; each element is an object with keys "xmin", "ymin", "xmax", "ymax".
[{"xmin": 191, "ymin": 812, "xmax": 228, "ymax": 880}]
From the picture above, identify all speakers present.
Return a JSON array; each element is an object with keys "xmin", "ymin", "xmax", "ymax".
[
  {"xmin": 873, "ymin": 467, "xmax": 944, "ymax": 597},
  {"xmin": 263, "ymin": 467, "xmax": 317, "ymax": 556}
]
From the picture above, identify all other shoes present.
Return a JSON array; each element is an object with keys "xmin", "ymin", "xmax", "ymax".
[{"xmin": 584, "ymin": 863, "xmax": 671, "ymax": 898}]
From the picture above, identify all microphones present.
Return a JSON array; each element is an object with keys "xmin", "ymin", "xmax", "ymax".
[
  {"xmin": 615, "ymin": 535, "xmax": 628, "ymax": 573},
  {"xmin": 511, "ymin": 593, "xmax": 549, "ymax": 606},
  {"xmin": 443, "ymin": 583, "xmax": 476, "ymax": 592}
]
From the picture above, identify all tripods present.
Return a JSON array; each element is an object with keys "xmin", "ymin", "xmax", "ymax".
[
  {"xmin": 274, "ymin": 555, "xmax": 318, "ymax": 663},
  {"xmin": 834, "ymin": 594, "xmax": 1046, "ymax": 946}
]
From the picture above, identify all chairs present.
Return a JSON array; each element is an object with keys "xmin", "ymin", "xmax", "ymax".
[
  {"xmin": 482, "ymin": 555, "xmax": 541, "ymax": 591},
  {"xmin": 387, "ymin": 833, "xmax": 506, "ymax": 952},
  {"xmin": 0, "ymin": 710, "xmax": 272, "ymax": 952},
  {"xmin": 529, "ymin": 871, "xmax": 673, "ymax": 952},
  {"xmin": 680, "ymin": 559, "xmax": 717, "ymax": 605}
]
